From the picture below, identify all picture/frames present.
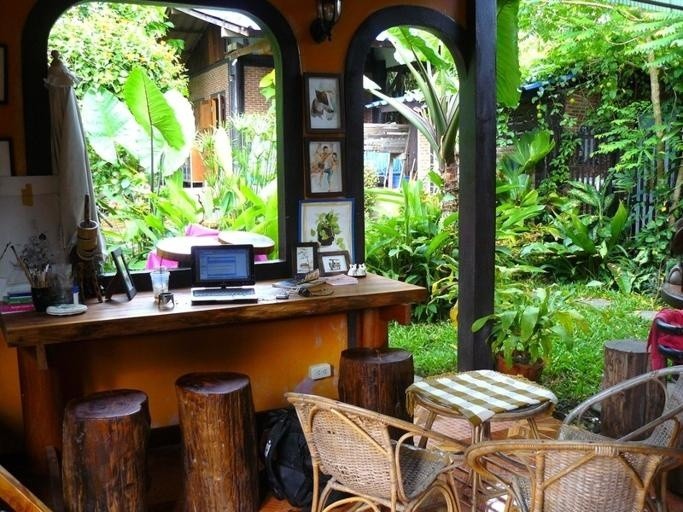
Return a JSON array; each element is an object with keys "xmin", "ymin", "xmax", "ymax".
[
  {"xmin": 289, "ymin": 70, "xmax": 356, "ymax": 278},
  {"xmin": 110, "ymin": 247, "xmax": 137, "ymax": 300}
]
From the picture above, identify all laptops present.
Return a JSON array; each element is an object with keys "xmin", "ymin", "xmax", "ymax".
[{"xmin": 191, "ymin": 244, "xmax": 258, "ymax": 304}]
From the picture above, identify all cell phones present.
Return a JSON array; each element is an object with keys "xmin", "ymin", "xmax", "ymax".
[{"xmin": 276, "ymin": 290, "xmax": 290, "ymax": 299}]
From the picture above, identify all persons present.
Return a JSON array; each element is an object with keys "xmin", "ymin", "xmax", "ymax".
[{"xmin": 311, "ymin": 143, "xmax": 340, "ymax": 193}]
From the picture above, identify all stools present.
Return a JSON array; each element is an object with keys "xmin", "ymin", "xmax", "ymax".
[
  {"xmin": 598, "ymin": 339, "xmax": 668, "ymax": 441},
  {"xmin": 59, "ymin": 370, "xmax": 262, "ymax": 510},
  {"xmin": 336, "ymin": 346, "xmax": 414, "ymax": 445}
]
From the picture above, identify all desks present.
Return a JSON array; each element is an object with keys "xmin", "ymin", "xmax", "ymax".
[{"xmin": 1, "ymin": 272, "xmax": 429, "ymax": 511}]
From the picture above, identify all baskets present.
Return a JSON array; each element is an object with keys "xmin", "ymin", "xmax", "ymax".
[{"xmin": 31, "ymin": 286, "xmax": 66, "ymax": 312}]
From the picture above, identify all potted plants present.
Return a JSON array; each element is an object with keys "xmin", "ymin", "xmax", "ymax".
[{"xmin": 470, "ymin": 282, "xmax": 591, "ymax": 383}]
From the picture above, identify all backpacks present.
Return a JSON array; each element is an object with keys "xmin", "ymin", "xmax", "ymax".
[{"xmin": 258, "ymin": 408, "xmax": 348, "ymax": 512}]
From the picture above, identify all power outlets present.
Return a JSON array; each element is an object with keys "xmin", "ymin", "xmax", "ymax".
[{"xmin": 306, "ymin": 363, "xmax": 331, "ymax": 381}]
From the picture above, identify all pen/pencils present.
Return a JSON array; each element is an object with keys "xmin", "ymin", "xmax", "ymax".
[
  {"xmin": 10, "ymin": 245, "xmax": 36, "ymax": 289},
  {"xmin": 28, "ymin": 264, "xmax": 50, "ymax": 288}
]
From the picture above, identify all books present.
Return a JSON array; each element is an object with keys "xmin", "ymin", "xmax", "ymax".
[{"xmin": 0, "ymin": 270, "xmax": 39, "ymax": 314}]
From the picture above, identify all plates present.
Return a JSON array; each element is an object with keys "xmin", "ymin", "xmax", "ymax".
[{"xmin": 45, "ymin": 304, "xmax": 89, "ymax": 316}]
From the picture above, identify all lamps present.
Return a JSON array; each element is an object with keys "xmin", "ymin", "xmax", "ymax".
[{"xmin": 310, "ymin": 0, "xmax": 341, "ymax": 42}]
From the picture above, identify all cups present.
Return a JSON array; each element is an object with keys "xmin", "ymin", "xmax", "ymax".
[
  {"xmin": 148, "ymin": 269, "xmax": 169, "ymax": 302},
  {"xmin": 30, "ymin": 286, "xmax": 52, "ymax": 310}
]
